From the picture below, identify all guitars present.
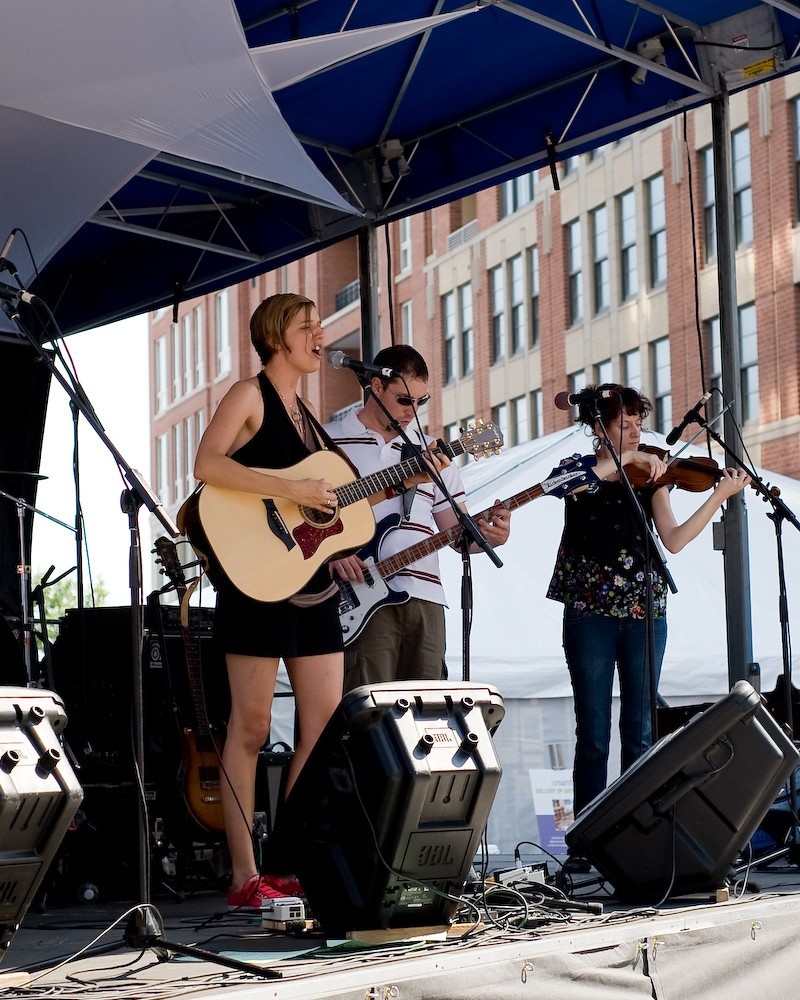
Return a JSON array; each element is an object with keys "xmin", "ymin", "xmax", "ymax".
[
  {"xmin": 341, "ymin": 452, "xmax": 603, "ymax": 648},
  {"xmin": 150, "ymin": 536, "xmax": 228, "ymax": 834},
  {"xmin": 186, "ymin": 414, "xmax": 505, "ymax": 609}
]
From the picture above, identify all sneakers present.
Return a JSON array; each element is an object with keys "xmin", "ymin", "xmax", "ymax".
[
  {"xmin": 260, "ymin": 874, "xmax": 312, "ymax": 910},
  {"xmin": 227, "ymin": 874, "xmax": 290, "ymax": 910}
]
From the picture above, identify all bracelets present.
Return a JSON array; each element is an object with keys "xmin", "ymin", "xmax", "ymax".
[
  {"xmin": 394, "ymin": 479, "xmax": 410, "ymax": 496},
  {"xmin": 383, "ymin": 486, "xmax": 394, "ymax": 500}
]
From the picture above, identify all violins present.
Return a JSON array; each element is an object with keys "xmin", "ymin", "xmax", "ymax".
[{"xmin": 622, "ymin": 442, "xmax": 782, "ymax": 501}]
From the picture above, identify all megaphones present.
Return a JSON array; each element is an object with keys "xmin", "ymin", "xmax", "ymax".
[
  {"xmin": 631, "ymin": 68, "xmax": 648, "ymax": 86},
  {"xmin": 656, "ymin": 54, "xmax": 669, "ymax": 69},
  {"xmin": 397, "ymin": 159, "xmax": 412, "ymax": 177},
  {"xmin": 381, "ymin": 165, "xmax": 393, "ymax": 182}
]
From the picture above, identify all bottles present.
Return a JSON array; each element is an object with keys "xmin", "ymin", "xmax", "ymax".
[{"xmin": 78, "ymin": 883, "xmax": 100, "ymax": 904}]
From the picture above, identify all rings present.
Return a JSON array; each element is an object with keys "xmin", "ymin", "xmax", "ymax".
[
  {"xmin": 324, "ymin": 500, "xmax": 332, "ymax": 508},
  {"xmin": 733, "ymin": 476, "xmax": 738, "ymax": 478}
]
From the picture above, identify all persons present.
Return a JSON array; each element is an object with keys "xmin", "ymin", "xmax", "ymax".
[
  {"xmin": 542, "ymin": 383, "xmax": 754, "ymax": 873},
  {"xmin": 322, "ymin": 344, "xmax": 512, "ymax": 685},
  {"xmin": 194, "ymin": 292, "xmax": 453, "ymax": 913}
]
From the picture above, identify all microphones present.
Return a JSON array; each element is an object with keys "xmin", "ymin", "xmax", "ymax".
[
  {"xmin": 554, "ymin": 388, "xmax": 614, "ymax": 410},
  {"xmin": 0, "ymin": 228, "xmax": 19, "ymax": 267},
  {"xmin": 327, "ymin": 350, "xmax": 400, "ymax": 380},
  {"xmin": 666, "ymin": 390, "xmax": 714, "ymax": 445},
  {"xmin": 0, "ymin": 282, "xmax": 47, "ymax": 307}
]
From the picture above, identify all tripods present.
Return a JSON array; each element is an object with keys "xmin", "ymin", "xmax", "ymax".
[{"xmin": 3, "ymin": 303, "xmax": 283, "ymax": 982}]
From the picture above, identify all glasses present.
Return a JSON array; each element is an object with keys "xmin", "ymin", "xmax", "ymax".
[{"xmin": 381, "ymin": 380, "xmax": 431, "ymax": 406}]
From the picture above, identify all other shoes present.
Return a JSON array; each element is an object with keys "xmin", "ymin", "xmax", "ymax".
[{"xmin": 562, "ymin": 855, "xmax": 591, "ymax": 873}]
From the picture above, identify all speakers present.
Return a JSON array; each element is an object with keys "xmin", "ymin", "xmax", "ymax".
[
  {"xmin": 277, "ymin": 679, "xmax": 506, "ymax": 934},
  {"xmin": 563, "ymin": 679, "xmax": 800, "ymax": 908},
  {"xmin": 0, "ymin": 685, "xmax": 85, "ymax": 964}
]
